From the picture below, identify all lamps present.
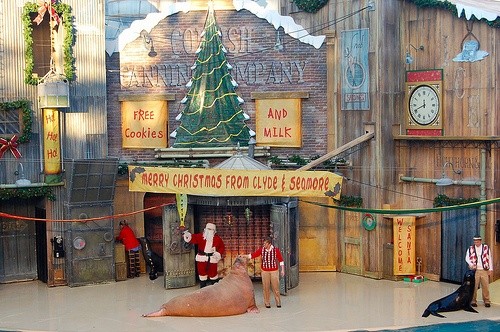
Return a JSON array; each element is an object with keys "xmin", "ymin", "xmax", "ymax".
[
  {"xmin": 274, "ymin": 31, "xmax": 284, "ymax": 51},
  {"xmin": 139, "ymin": 29, "xmax": 157, "ymax": 57},
  {"xmin": 14, "ymin": 162, "xmax": 29, "ymax": 185},
  {"xmin": 405, "ymin": 44, "xmax": 423, "ymax": 64},
  {"xmin": 435, "ymin": 162, "xmax": 463, "ymax": 186}
]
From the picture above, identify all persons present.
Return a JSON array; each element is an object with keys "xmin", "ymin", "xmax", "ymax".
[
  {"xmin": 465, "ymin": 234, "xmax": 493, "ymax": 308},
  {"xmin": 240, "ymin": 237, "xmax": 285, "ymax": 308},
  {"xmin": 114, "ymin": 219, "xmax": 141, "ymax": 279},
  {"xmin": 183, "ymin": 222, "xmax": 224, "ymax": 288}
]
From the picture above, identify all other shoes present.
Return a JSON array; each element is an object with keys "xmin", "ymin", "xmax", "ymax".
[
  {"xmin": 135, "ymin": 273, "xmax": 140, "ymax": 277},
  {"xmin": 266, "ymin": 306, "xmax": 271, "ymax": 308},
  {"xmin": 277, "ymin": 305, "xmax": 281, "ymax": 308},
  {"xmin": 485, "ymin": 303, "xmax": 490, "ymax": 307},
  {"xmin": 471, "ymin": 304, "xmax": 477, "ymax": 307},
  {"xmin": 127, "ymin": 274, "xmax": 135, "ymax": 278}
]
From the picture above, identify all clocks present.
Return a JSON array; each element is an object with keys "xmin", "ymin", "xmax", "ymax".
[{"xmin": 408, "ymin": 84, "xmax": 440, "ymax": 125}]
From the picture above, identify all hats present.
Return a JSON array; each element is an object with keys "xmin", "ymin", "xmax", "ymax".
[{"xmin": 473, "ymin": 234, "xmax": 482, "ymax": 239}]
